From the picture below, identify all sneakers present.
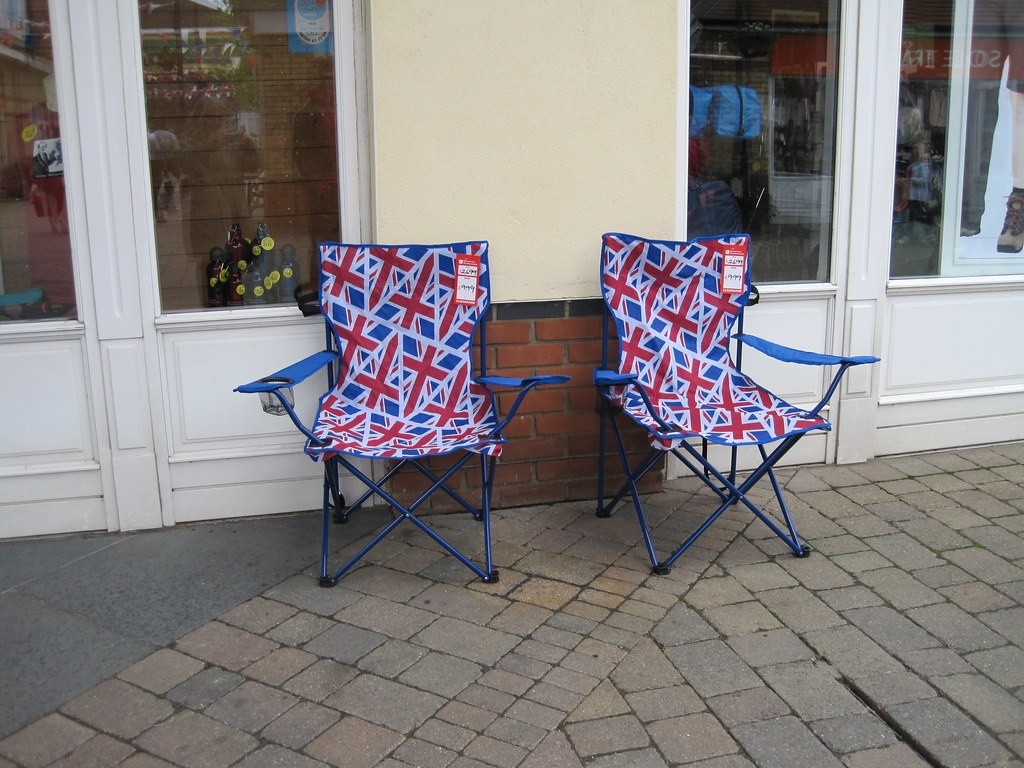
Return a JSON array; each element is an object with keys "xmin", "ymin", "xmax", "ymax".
[{"xmin": 996, "ymin": 192, "xmax": 1024, "ymax": 252}]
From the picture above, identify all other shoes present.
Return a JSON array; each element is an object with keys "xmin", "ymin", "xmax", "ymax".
[
  {"xmin": 156, "ymin": 218, "xmax": 166, "ymax": 222},
  {"xmin": 59, "ymin": 217, "xmax": 67, "ymax": 233},
  {"xmin": 50, "ymin": 217, "xmax": 57, "ymax": 233}
]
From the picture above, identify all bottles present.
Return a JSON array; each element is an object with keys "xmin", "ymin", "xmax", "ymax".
[{"xmin": 205, "ymin": 223, "xmax": 299, "ymax": 307}]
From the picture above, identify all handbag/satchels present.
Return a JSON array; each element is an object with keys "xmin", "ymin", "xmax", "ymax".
[
  {"xmin": 164, "ymin": 177, "xmax": 174, "ymax": 196},
  {"xmin": 34, "ymin": 192, "xmax": 45, "ymax": 218}
]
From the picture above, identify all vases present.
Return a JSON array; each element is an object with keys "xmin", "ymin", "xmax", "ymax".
[{"xmin": 735, "ymin": 35, "xmax": 771, "ymax": 58}]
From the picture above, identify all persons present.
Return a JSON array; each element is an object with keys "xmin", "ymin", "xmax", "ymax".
[
  {"xmin": 13, "ymin": 120, "xmax": 69, "ymax": 235},
  {"xmin": 146, "ymin": 94, "xmax": 260, "ymax": 307},
  {"xmin": 959, "ymin": 0, "xmax": 1024, "ymax": 253},
  {"xmin": 899, "ymin": 149, "xmax": 939, "ymax": 243},
  {"xmin": 687, "ymin": 114, "xmax": 741, "ymax": 245},
  {"xmin": 294, "ymin": 58, "xmax": 341, "ymax": 284}
]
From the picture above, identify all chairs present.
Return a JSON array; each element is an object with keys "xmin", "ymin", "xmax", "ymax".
[
  {"xmin": 233, "ymin": 240, "xmax": 572, "ymax": 588},
  {"xmin": 591, "ymin": 231, "xmax": 883, "ymax": 576}
]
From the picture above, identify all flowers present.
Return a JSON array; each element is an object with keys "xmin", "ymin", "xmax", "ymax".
[{"xmin": 732, "ymin": 21, "xmax": 778, "ymax": 42}]
